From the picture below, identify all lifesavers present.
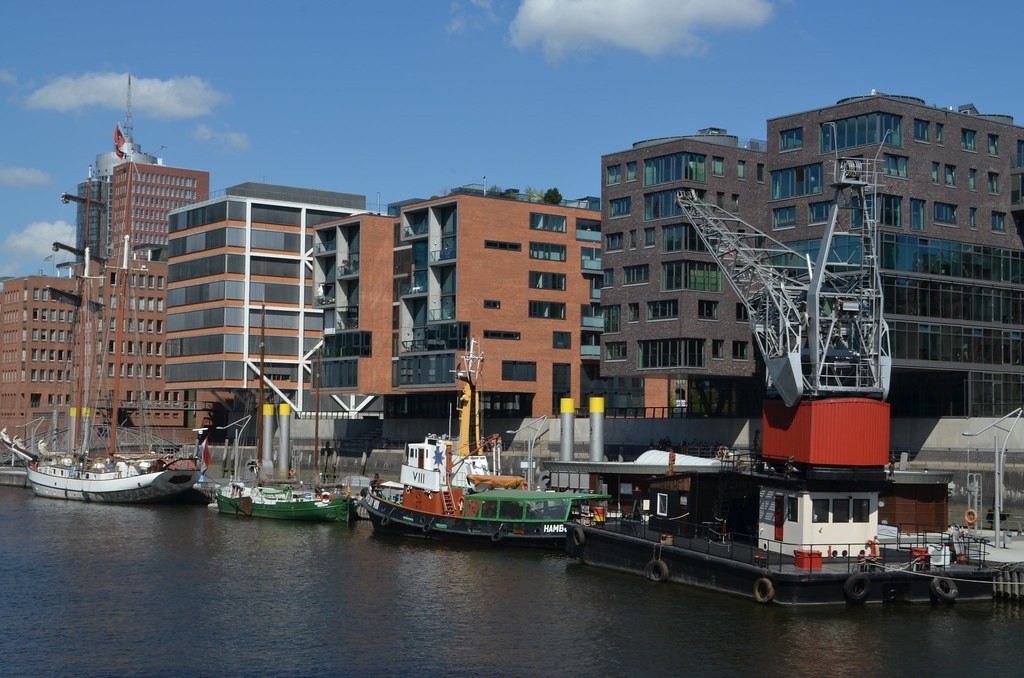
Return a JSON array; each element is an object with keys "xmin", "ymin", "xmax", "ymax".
[
  {"xmin": 844, "ymin": 574, "xmax": 870, "ymax": 603},
  {"xmin": 320, "ymin": 492, "xmax": 331, "ymax": 504},
  {"xmin": 646, "ymin": 559, "xmax": 669, "ymax": 581},
  {"xmin": 754, "ymin": 577, "xmax": 773, "ymax": 603},
  {"xmin": 423, "ymin": 523, "xmax": 431, "ymax": 533},
  {"xmin": 289, "ymin": 468, "xmax": 295, "ymax": 477},
  {"xmin": 931, "ymin": 574, "xmax": 958, "ymax": 601},
  {"xmin": 964, "ymin": 508, "xmax": 977, "ymax": 523},
  {"xmin": 381, "ymin": 515, "xmax": 390, "ymax": 527},
  {"xmin": 491, "ymin": 531, "xmax": 503, "ymax": 542},
  {"xmin": 573, "ymin": 527, "xmax": 585, "ymax": 546}
]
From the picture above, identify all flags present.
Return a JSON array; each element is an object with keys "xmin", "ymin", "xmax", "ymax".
[
  {"xmin": 289, "ymin": 466, "xmax": 296, "ymax": 476},
  {"xmin": 198, "ymin": 435, "xmax": 211, "ymax": 473},
  {"xmin": 113, "ymin": 123, "xmax": 126, "ymax": 160}
]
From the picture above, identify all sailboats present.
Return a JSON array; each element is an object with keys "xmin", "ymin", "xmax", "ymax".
[{"xmin": 0, "ymin": 153, "xmax": 204, "ymax": 503}]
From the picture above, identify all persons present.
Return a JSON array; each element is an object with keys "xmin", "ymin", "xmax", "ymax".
[{"xmin": 369, "ymin": 472, "xmax": 379, "ymax": 495}]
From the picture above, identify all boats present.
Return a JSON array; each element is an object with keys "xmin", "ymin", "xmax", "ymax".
[
  {"xmin": 360, "ymin": 337, "xmax": 613, "ymax": 547},
  {"xmin": 213, "ymin": 479, "xmax": 354, "ymax": 521}
]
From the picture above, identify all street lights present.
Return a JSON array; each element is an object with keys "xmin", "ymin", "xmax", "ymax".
[
  {"xmin": 216, "ymin": 415, "xmax": 252, "ymax": 481},
  {"xmin": 16, "ymin": 416, "xmax": 45, "ymax": 451},
  {"xmin": 506, "ymin": 414, "xmax": 549, "ymax": 490},
  {"xmin": 961, "ymin": 407, "xmax": 1023, "ymax": 547}
]
